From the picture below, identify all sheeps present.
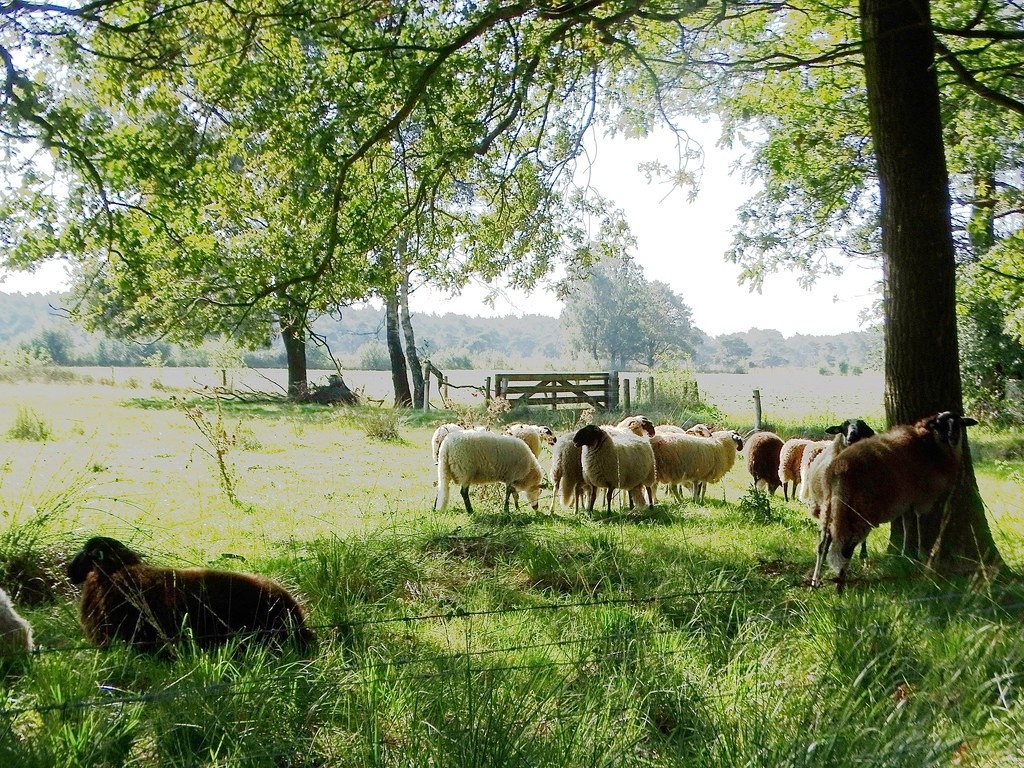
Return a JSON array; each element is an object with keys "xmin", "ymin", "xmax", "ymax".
[
  {"xmin": 744, "ymin": 411, "xmax": 977, "ymax": 590},
  {"xmin": 1, "ymin": 589, "xmax": 33, "ymax": 664},
  {"xmin": 432, "ymin": 423, "xmax": 556, "ymax": 517},
  {"xmin": 552, "ymin": 415, "xmax": 744, "ymax": 518},
  {"xmin": 68, "ymin": 536, "xmax": 317, "ymax": 658}
]
